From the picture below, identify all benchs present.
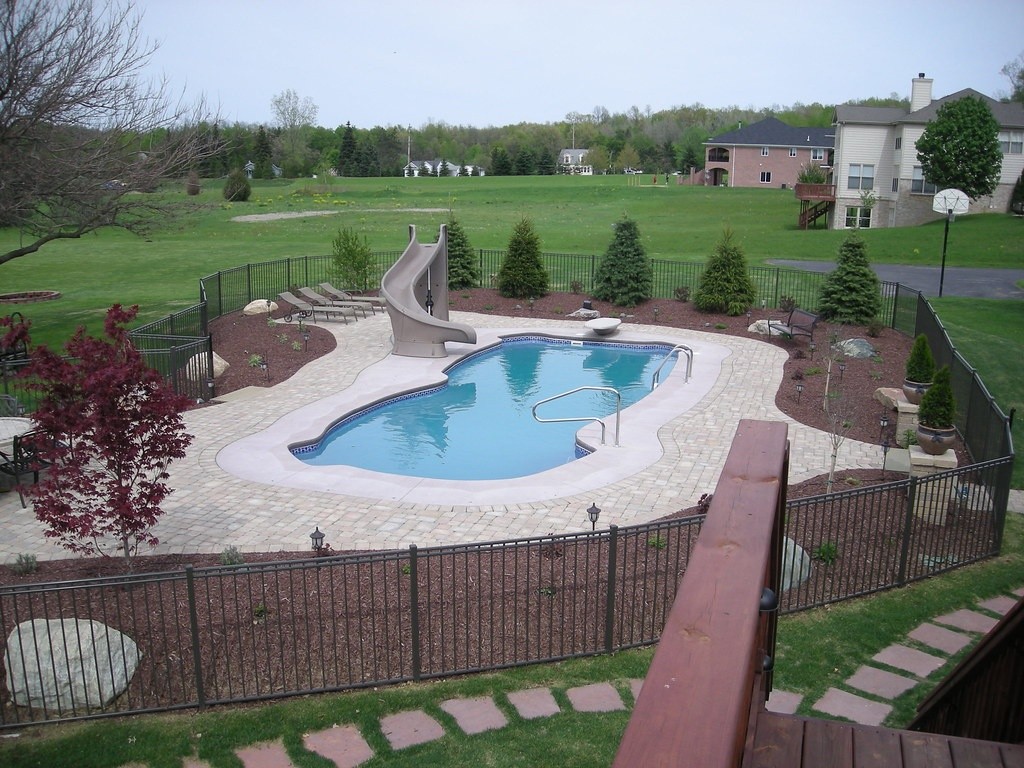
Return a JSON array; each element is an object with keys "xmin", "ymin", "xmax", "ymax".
[{"xmin": 768, "ymin": 308, "xmax": 820, "ymax": 350}]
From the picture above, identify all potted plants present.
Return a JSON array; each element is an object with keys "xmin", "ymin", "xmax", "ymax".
[
  {"xmin": 902, "ymin": 332, "xmax": 938, "ymax": 405},
  {"xmin": 916, "ymin": 363, "xmax": 957, "ymax": 455}
]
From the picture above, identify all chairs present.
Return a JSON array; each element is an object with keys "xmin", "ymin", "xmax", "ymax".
[
  {"xmin": 0, "ymin": 394, "xmax": 17, "ymax": 417},
  {"xmin": 317, "ymin": 282, "xmax": 387, "ymax": 313},
  {"xmin": 277, "ymin": 292, "xmax": 358, "ymax": 325},
  {"xmin": 298, "ymin": 287, "xmax": 375, "ymax": 319},
  {"xmin": 0, "ymin": 429, "xmax": 74, "ymax": 508}
]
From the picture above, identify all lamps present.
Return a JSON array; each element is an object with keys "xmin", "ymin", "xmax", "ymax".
[
  {"xmin": 303, "ymin": 330, "xmax": 309, "ymax": 342},
  {"xmin": 795, "ymin": 381, "xmax": 804, "ymax": 393},
  {"xmin": 880, "ymin": 415, "xmax": 889, "ymax": 430},
  {"xmin": 259, "ymin": 357, "xmax": 268, "ymax": 370},
  {"xmin": 809, "ymin": 340, "xmax": 817, "ymax": 350},
  {"xmin": 16, "ymin": 399, "xmax": 26, "ymax": 416},
  {"xmin": 266, "ymin": 298, "xmax": 271, "ymax": 307},
  {"xmin": 746, "ymin": 309, "xmax": 752, "ymax": 318},
  {"xmin": 529, "ymin": 296, "xmax": 534, "ymax": 305},
  {"xmin": 839, "ymin": 362, "xmax": 846, "ymax": 372},
  {"xmin": 586, "ymin": 503, "xmax": 601, "ymax": 523},
  {"xmin": 310, "ymin": 526, "xmax": 325, "ymax": 550},
  {"xmin": 653, "ymin": 305, "xmax": 659, "ymax": 315}
]
